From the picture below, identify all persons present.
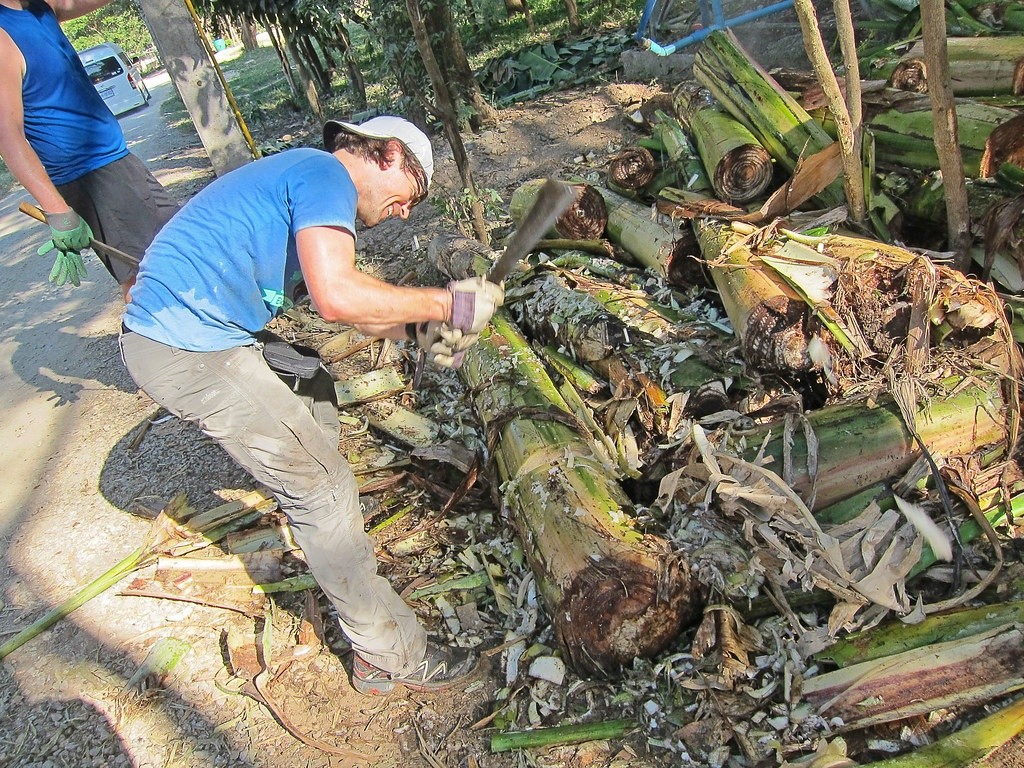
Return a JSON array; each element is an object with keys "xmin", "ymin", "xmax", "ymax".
[
  {"xmin": 120, "ymin": 115, "xmax": 504, "ymax": 697},
  {"xmin": 0, "ymin": 0, "xmax": 179, "ymax": 296}
]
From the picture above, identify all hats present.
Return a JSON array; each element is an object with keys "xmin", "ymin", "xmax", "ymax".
[{"xmin": 322, "ymin": 114, "xmax": 434, "ymax": 204}]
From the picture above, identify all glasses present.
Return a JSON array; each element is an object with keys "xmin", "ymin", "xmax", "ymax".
[{"xmin": 398, "ymin": 141, "xmax": 429, "ymax": 210}]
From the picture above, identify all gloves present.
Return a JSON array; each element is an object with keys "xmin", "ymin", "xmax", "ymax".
[
  {"xmin": 42, "ymin": 207, "xmax": 95, "ymax": 253},
  {"xmin": 38, "ymin": 239, "xmax": 89, "ymax": 287},
  {"xmin": 445, "ymin": 277, "xmax": 506, "ymax": 335},
  {"xmin": 404, "ymin": 317, "xmax": 483, "ymax": 369}
]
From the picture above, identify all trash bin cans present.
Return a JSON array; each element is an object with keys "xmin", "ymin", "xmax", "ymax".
[{"xmin": 212, "ymin": 34, "xmax": 226, "ymax": 52}]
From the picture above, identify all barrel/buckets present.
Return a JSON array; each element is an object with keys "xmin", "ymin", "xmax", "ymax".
[{"xmin": 213, "ymin": 39, "xmax": 225, "ymax": 52}]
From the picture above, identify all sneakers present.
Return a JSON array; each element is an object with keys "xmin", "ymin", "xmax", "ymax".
[
  {"xmin": 362, "ymin": 496, "xmax": 377, "ymax": 515},
  {"xmin": 352, "ymin": 635, "xmax": 480, "ymax": 696}
]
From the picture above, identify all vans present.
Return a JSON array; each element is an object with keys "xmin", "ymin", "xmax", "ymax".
[{"xmin": 78, "ymin": 41, "xmax": 151, "ymax": 117}]
